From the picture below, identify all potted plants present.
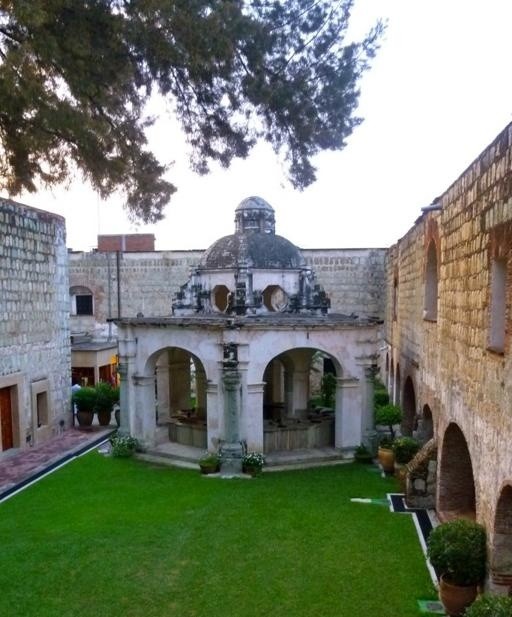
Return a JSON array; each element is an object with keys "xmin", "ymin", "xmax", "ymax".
[
  {"xmin": 73, "ymin": 381, "xmax": 120, "ymax": 427},
  {"xmin": 425, "ymin": 519, "xmax": 487, "ymax": 617},
  {"xmin": 198, "ymin": 451, "xmax": 220, "ymax": 474},
  {"xmin": 394, "ymin": 436, "xmax": 418, "ymax": 479},
  {"xmin": 379, "ymin": 439, "xmax": 395, "ymax": 472},
  {"xmin": 355, "ymin": 443, "xmax": 373, "ymax": 463}
]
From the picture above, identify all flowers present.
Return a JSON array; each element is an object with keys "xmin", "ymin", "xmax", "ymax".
[
  {"xmin": 110, "ymin": 436, "xmax": 137, "ymax": 456},
  {"xmin": 243, "ymin": 452, "xmax": 264, "ymax": 465}
]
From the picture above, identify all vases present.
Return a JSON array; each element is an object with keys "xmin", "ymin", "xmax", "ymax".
[{"xmin": 243, "ymin": 466, "xmax": 262, "ymax": 474}]
